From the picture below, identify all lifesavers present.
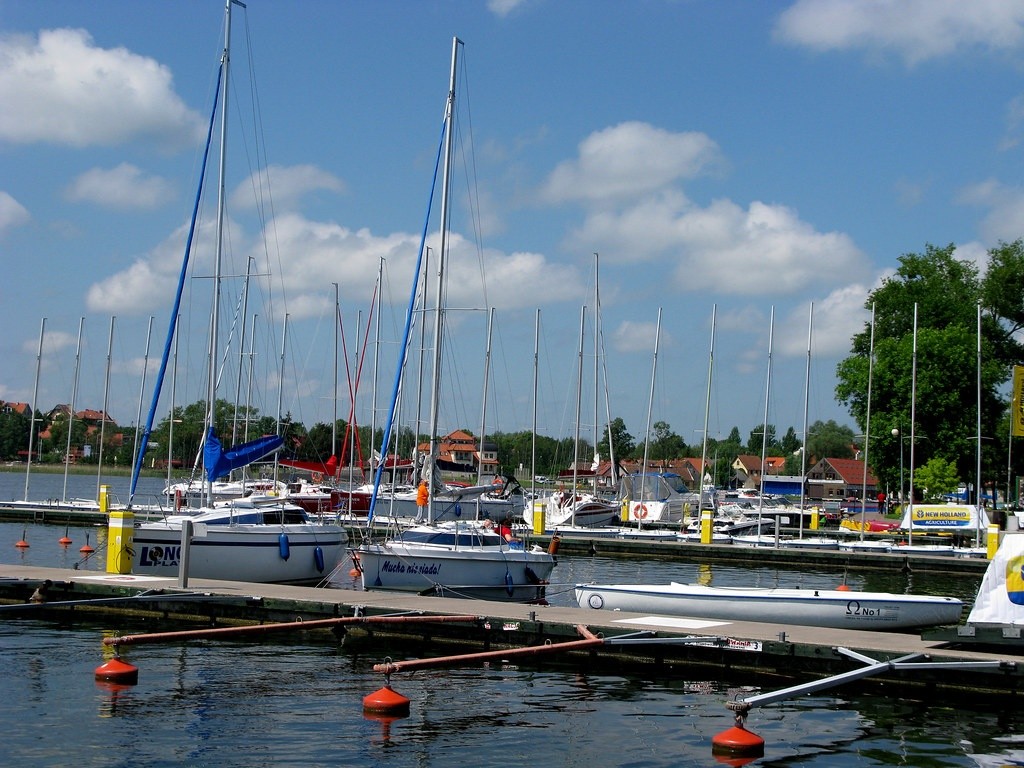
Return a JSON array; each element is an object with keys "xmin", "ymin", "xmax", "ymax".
[
  {"xmin": 634, "ymin": 504, "xmax": 647, "ymax": 519},
  {"xmin": 312, "ymin": 471, "xmax": 323, "ymax": 482},
  {"xmin": 492, "ymin": 478, "xmax": 503, "ymax": 493}
]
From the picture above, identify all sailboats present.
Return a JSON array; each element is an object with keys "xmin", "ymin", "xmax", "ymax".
[
  {"xmin": 0, "ymin": 250, "xmax": 1024, "ymax": 575},
  {"xmin": 344, "ymin": 36, "xmax": 558, "ymax": 592},
  {"xmin": 126, "ymin": 2, "xmax": 357, "ymax": 578}
]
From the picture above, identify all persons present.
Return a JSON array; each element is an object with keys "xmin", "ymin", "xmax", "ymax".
[
  {"xmin": 414, "ymin": 480, "xmax": 429, "ymax": 523},
  {"xmin": 495, "ymin": 518, "xmax": 522, "ymax": 543},
  {"xmin": 877, "ymin": 490, "xmax": 905, "ymax": 514}
]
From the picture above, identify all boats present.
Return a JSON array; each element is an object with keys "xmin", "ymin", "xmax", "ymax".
[{"xmin": 573, "ymin": 583, "xmax": 963, "ymax": 634}]
